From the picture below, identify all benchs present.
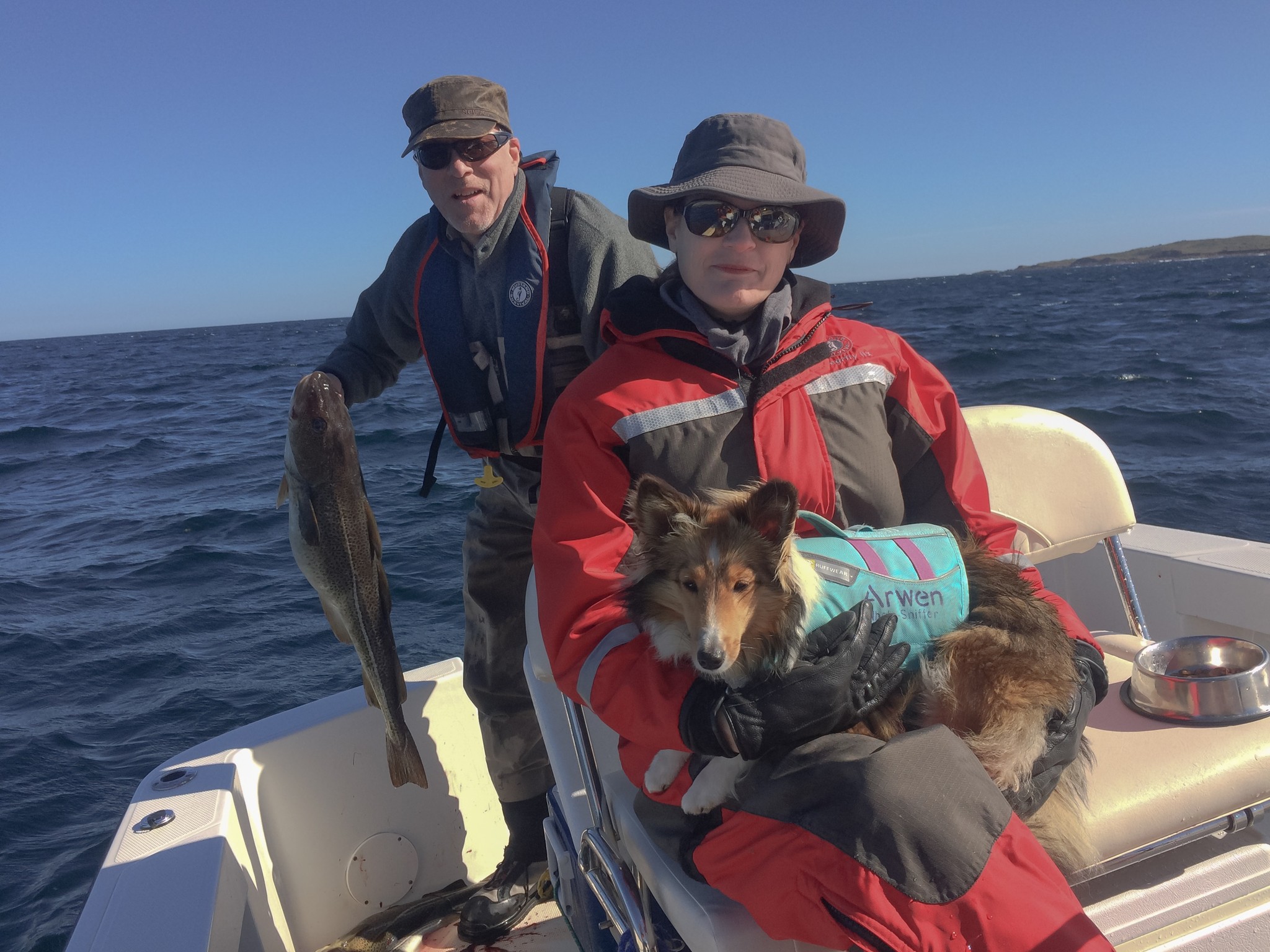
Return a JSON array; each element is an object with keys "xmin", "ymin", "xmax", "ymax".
[{"xmin": 523, "ymin": 405, "xmax": 1270, "ymax": 952}]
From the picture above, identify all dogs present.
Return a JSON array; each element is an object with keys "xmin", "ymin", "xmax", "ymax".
[{"xmin": 634, "ymin": 468, "xmax": 1106, "ymax": 872}]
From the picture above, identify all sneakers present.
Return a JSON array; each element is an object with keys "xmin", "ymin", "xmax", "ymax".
[{"xmin": 457, "ymin": 846, "xmax": 554, "ymax": 945}]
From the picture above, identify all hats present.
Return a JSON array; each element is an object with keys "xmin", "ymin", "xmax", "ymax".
[
  {"xmin": 401, "ymin": 75, "xmax": 513, "ymax": 158},
  {"xmin": 628, "ymin": 114, "xmax": 847, "ymax": 268}
]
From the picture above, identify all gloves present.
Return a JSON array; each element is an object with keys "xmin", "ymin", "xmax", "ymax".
[
  {"xmin": 1002, "ymin": 644, "xmax": 1109, "ymax": 821},
  {"xmin": 720, "ymin": 600, "xmax": 910, "ymax": 762}
]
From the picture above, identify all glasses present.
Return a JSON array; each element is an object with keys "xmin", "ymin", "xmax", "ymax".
[
  {"xmin": 413, "ymin": 132, "xmax": 512, "ymax": 170},
  {"xmin": 675, "ymin": 199, "xmax": 802, "ymax": 243}
]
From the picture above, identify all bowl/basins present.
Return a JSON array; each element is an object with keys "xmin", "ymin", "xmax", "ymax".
[{"xmin": 1133, "ymin": 634, "xmax": 1270, "ymax": 722}]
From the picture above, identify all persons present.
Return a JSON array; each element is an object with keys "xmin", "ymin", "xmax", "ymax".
[
  {"xmin": 312, "ymin": 60, "xmax": 664, "ymax": 945},
  {"xmin": 529, "ymin": 114, "xmax": 1126, "ymax": 952}
]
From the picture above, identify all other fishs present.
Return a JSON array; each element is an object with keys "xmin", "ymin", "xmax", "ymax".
[{"xmin": 276, "ymin": 368, "xmax": 433, "ymax": 790}]
